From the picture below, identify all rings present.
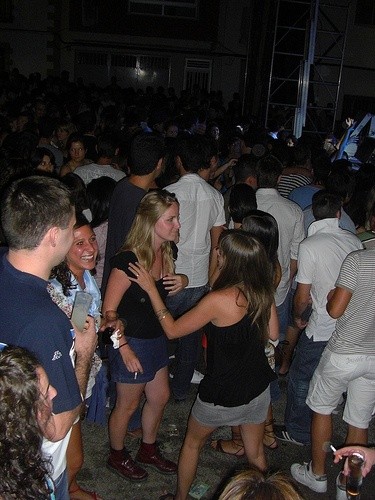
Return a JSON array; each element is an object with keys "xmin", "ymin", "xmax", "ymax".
[{"xmin": 136, "ymin": 274, "xmax": 139, "ymax": 277}]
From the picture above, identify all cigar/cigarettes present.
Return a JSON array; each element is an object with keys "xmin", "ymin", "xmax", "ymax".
[
  {"xmin": 330, "ymin": 444, "xmax": 343, "ymax": 460},
  {"xmin": 134, "ymin": 372, "xmax": 138, "ymax": 380}
]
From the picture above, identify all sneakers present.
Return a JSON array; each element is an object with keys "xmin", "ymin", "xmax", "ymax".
[
  {"xmin": 335, "ymin": 471, "xmax": 347, "ymax": 500},
  {"xmin": 274, "ymin": 425, "xmax": 305, "ymax": 446},
  {"xmin": 291, "ymin": 460, "xmax": 328, "ymax": 493}
]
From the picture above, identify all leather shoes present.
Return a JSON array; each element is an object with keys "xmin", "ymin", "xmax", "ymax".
[
  {"xmin": 134, "ymin": 439, "xmax": 177, "ymax": 475},
  {"xmin": 107, "ymin": 447, "xmax": 149, "ymax": 482}
]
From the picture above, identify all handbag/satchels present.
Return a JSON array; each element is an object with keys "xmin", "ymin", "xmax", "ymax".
[{"xmin": 264, "ymin": 336, "xmax": 289, "ymax": 375}]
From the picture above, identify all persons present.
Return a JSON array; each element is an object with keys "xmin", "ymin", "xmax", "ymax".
[
  {"xmin": 0, "ymin": 345, "xmax": 59, "ymax": 500},
  {"xmin": 290, "ymin": 236, "xmax": 375, "ymax": 493},
  {"xmin": 333, "ymin": 445, "xmax": 375, "ymax": 477},
  {"xmin": 100, "ymin": 188, "xmax": 189, "ymax": 483},
  {"xmin": 216, "ymin": 464, "xmax": 304, "ymax": 500},
  {"xmin": 45, "ymin": 212, "xmax": 128, "ymax": 500},
  {"xmin": 0, "ymin": 66, "xmax": 375, "ymax": 414},
  {"xmin": 1, "ymin": 175, "xmax": 98, "ymax": 500},
  {"xmin": 208, "ymin": 209, "xmax": 283, "ymax": 458},
  {"xmin": 124, "ymin": 229, "xmax": 280, "ymax": 500},
  {"xmin": 271, "ymin": 187, "xmax": 367, "ymax": 446}
]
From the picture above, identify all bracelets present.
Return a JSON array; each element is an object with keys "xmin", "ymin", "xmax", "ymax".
[
  {"xmin": 117, "ymin": 342, "xmax": 128, "ymax": 350},
  {"xmin": 154, "ymin": 308, "xmax": 170, "ymax": 322}
]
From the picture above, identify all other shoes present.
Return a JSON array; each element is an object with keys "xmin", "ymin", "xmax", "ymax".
[
  {"xmin": 169, "ymin": 368, "xmax": 204, "ymax": 384},
  {"xmin": 127, "ymin": 428, "xmax": 143, "ymax": 437}
]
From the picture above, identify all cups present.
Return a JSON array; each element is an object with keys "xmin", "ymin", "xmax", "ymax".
[
  {"xmin": 345, "ymin": 448, "xmax": 366, "ymax": 500},
  {"xmin": 71, "ymin": 289, "xmax": 92, "ymax": 332},
  {"xmin": 101, "ymin": 311, "xmax": 119, "ymax": 344}
]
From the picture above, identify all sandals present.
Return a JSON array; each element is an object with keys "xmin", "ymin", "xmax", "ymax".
[
  {"xmin": 263, "ymin": 422, "xmax": 279, "ymax": 448},
  {"xmin": 211, "ymin": 431, "xmax": 245, "ymax": 457},
  {"xmin": 70, "ymin": 486, "xmax": 104, "ymax": 500}
]
